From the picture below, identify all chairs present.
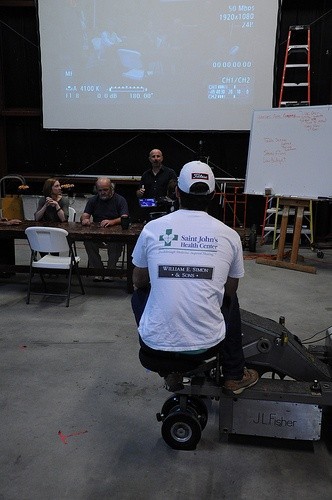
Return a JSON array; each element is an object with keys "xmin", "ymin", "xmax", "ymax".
[
  {"xmin": 68, "ymin": 207, "xmax": 77, "ymax": 258},
  {"xmin": 25, "ymin": 226, "xmax": 85, "ymax": 307}
]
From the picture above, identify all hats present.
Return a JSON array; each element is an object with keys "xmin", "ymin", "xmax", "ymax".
[{"xmin": 177, "ymin": 160, "xmax": 215, "ymax": 196}]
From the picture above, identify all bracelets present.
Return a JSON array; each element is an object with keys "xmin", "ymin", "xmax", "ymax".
[{"xmin": 57, "ymin": 207, "xmax": 62, "ymax": 212}]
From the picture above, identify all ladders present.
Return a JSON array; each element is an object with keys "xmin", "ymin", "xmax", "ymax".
[{"xmin": 260, "ymin": 24, "xmax": 314, "ymax": 245}]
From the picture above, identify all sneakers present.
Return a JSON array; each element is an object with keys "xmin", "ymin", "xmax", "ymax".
[
  {"xmin": 222, "ymin": 367, "xmax": 259, "ymax": 395},
  {"xmin": 164, "ymin": 374, "xmax": 184, "ymax": 391}
]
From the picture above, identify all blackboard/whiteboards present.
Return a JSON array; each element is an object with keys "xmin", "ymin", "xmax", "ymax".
[{"xmin": 243, "ymin": 104, "xmax": 332, "ymax": 200}]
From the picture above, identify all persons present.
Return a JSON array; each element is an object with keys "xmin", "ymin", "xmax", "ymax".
[
  {"xmin": 138, "ymin": 149, "xmax": 179, "ymax": 223},
  {"xmin": 34, "ymin": 178, "xmax": 69, "ymax": 277},
  {"xmin": 131, "ymin": 161, "xmax": 259, "ymax": 394},
  {"xmin": 0, "ymin": 219, "xmax": 22, "ymax": 225},
  {"xmin": 80, "ymin": 177, "xmax": 128, "ymax": 281}
]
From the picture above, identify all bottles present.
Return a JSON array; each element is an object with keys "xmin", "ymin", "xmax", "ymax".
[
  {"xmin": 279, "ymin": 315, "xmax": 288, "ymax": 346},
  {"xmin": 310, "ymin": 380, "xmax": 322, "ymax": 396},
  {"xmin": 237, "ymin": 223, "xmax": 257, "ymax": 252}
]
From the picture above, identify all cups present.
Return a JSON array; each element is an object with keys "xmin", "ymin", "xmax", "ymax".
[{"xmin": 121, "ymin": 215, "xmax": 129, "ymax": 230}]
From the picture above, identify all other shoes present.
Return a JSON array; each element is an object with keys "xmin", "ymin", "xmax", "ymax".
[
  {"xmin": 103, "ymin": 277, "xmax": 114, "ymax": 281},
  {"xmin": 94, "ymin": 276, "xmax": 103, "ymax": 281},
  {"xmin": 43, "ymin": 274, "xmax": 50, "ymax": 279},
  {"xmin": 50, "ymin": 274, "xmax": 58, "ymax": 279}
]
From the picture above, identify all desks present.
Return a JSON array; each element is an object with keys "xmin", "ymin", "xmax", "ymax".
[{"xmin": 0, "ymin": 220, "xmax": 144, "ymax": 294}]
